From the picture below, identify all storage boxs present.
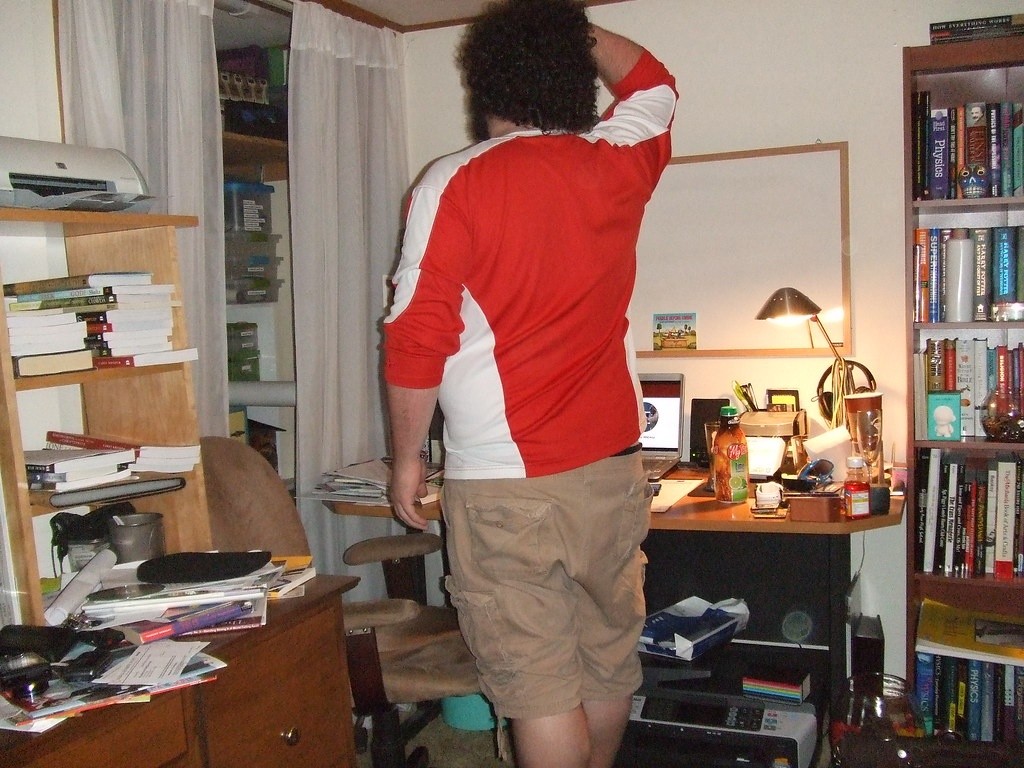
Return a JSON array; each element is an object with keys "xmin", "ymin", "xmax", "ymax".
[
  {"xmin": 787, "ymin": 496, "xmax": 841, "ymax": 524},
  {"xmin": 637, "ymin": 596, "xmax": 751, "ymax": 662},
  {"xmin": 224, "ymin": 181, "xmax": 275, "ymax": 234},
  {"xmin": 225, "ymin": 255, "xmax": 283, "ymax": 279},
  {"xmin": 226, "ymin": 322, "xmax": 258, "ymax": 338},
  {"xmin": 225, "ymin": 231, "xmax": 282, "ymax": 257},
  {"xmin": 228, "ymin": 358, "xmax": 260, "ymax": 381},
  {"xmin": 228, "ymin": 338, "xmax": 261, "ymax": 362},
  {"xmin": 225, "ymin": 278, "xmax": 285, "ymax": 302}
]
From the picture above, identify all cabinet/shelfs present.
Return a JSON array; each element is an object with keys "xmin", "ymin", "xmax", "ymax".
[
  {"xmin": 0, "ymin": 206, "xmax": 213, "ymax": 626},
  {"xmin": 0, "ymin": 575, "xmax": 362, "ymax": 768},
  {"xmin": 902, "ymin": 36, "xmax": 1024, "ymax": 726}
]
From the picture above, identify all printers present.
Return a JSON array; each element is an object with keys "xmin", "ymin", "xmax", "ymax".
[{"xmin": 613, "ymin": 696, "xmax": 818, "ymax": 768}]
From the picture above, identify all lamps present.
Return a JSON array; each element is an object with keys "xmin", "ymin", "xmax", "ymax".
[{"xmin": 755, "ymin": 287, "xmax": 855, "ymax": 394}]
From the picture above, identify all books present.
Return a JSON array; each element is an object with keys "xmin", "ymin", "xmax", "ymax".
[
  {"xmin": 1, "ymin": 272, "xmax": 200, "ymax": 380},
  {"xmin": 915, "ymin": 448, "xmax": 1024, "ymax": 580},
  {"xmin": 18, "ymin": 431, "xmax": 203, "ymax": 512},
  {"xmin": 1, "ymin": 550, "xmax": 317, "ymax": 735},
  {"xmin": 915, "ymin": 598, "xmax": 1024, "ymax": 747},
  {"xmin": 910, "ymin": 89, "xmax": 1024, "ymax": 201},
  {"xmin": 914, "ymin": 337, "xmax": 1024, "ymax": 441},
  {"xmin": 312, "ymin": 458, "xmax": 446, "ymax": 507},
  {"xmin": 913, "ymin": 225, "xmax": 1024, "ymax": 324}
]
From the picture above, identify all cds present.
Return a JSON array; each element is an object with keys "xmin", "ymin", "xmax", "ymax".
[{"xmin": 86, "ymin": 584, "xmax": 166, "ymax": 601}]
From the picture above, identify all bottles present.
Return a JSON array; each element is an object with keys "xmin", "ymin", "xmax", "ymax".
[
  {"xmin": 713, "ymin": 406, "xmax": 750, "ymax": 503},
  {"xmin": 844, "ymin": 457, "xmax": 871, "ymax": 522}
]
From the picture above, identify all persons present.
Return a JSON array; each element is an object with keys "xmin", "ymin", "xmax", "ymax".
[{"xmin": 383, "ymin": 0, "xmax": 681, "ymax": 768}]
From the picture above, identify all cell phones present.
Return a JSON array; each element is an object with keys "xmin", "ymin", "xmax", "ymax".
[
  {"xmin": 651, "ymin": 484, "xmax": 661, "ymax": 496},
  {"xmin": 65, "ymin": 648, "xmax": 113, "ymax": 682}
]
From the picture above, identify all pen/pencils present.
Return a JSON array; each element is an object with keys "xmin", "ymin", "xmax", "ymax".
[{"xmin": 733, "ymin": 381, "xmax": 759, "ymax": 412}]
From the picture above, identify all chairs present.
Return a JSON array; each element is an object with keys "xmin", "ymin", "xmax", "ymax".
[{"xmin": 199, "ymin": 435, "xmax": 482, "ymax": 768}]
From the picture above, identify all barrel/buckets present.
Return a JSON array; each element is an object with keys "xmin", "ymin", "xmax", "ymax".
[{"xmin": 441, "ymin": 694, "xmax": 508, "ymax": 730}]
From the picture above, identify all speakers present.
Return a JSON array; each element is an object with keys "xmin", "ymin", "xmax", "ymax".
[{"xmin": 690, "ymin": 398, "xmax": 730, "ymax": 468}]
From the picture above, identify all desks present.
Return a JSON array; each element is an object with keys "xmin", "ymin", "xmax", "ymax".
[{"xmin": 322, "ymin": 462, "xmax": 906, "ymax": 724}]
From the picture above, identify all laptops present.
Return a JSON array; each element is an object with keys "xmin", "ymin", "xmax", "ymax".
[{"xmin": 638, "ymin": 373, "xmax": 686, "ymax": 480}]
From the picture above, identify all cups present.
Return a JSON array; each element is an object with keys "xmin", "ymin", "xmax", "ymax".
[
  {"xmin": 844, "ymin": 391, "xmax": 884, "ymax": 453},
  {"xmin": 871, "ymin": 486, "xmax": 890, "ymax": 515},
  {"xmin": 756, "ymin": 482, "xmax": 784, "ymax": 508},
  {"xmin": 108, "ymin": 513, "xmax": 167, "ymax": 564}
]
